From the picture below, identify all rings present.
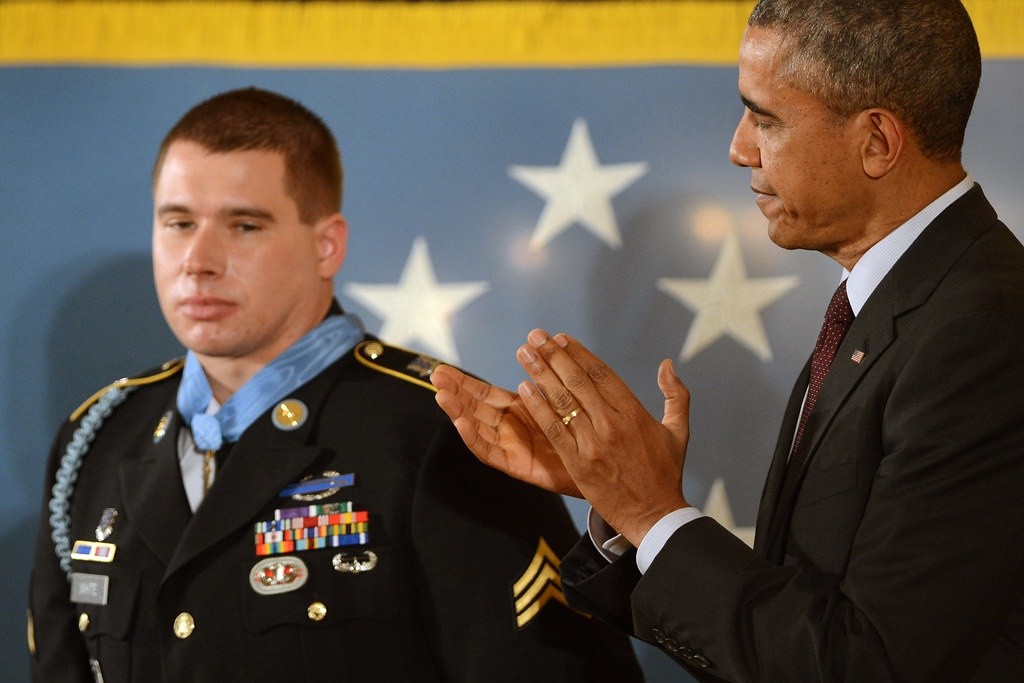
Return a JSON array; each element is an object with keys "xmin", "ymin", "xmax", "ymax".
[{"xmin": 562, "ymin": 407, "xmax": 583, "ymax": 426}]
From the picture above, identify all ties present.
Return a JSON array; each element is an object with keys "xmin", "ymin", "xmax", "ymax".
[{"xmin": 789, "ymin": 278, "xmax": 852, "ymax": 456}]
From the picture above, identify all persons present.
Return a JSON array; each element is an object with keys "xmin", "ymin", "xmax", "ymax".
[
  {"xmin": 25, "ymin": 89, "xmax": 646, "ymax": 682},
  {"xmin": 430, "ymin": 1, "xmax": 1024, "ymax": 683}
]
